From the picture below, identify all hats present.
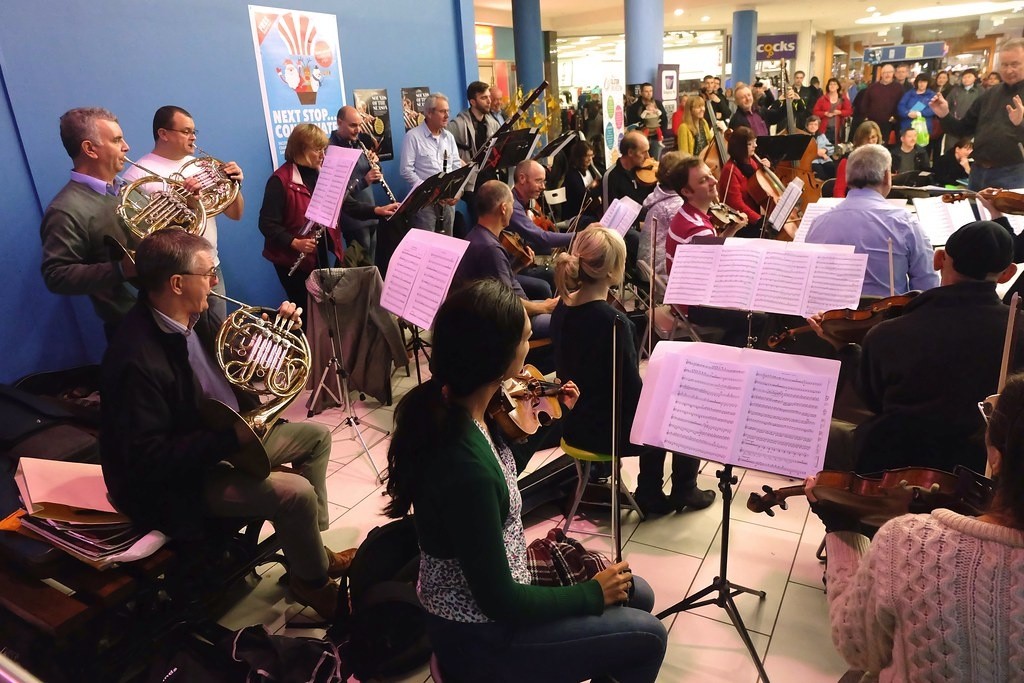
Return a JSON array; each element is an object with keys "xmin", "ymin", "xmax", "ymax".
[{"xmin": 945, "ymin": 220, "xmax": 1013, "ymax": 280}]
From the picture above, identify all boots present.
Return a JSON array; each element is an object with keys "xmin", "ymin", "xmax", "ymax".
[
  {"xmin": 633, "ymin": 472, "xmax": 673, "ymax": 517},
  {"xmin": 668, "ymin": 472, "xmax": 716, "ymax": 514}
]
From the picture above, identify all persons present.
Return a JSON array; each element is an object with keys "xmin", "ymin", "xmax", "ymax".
[
  {"xmin": 42, "ymin": 108, "xmax": 201, "ymax": 342},
  {"xmin": 804, "ymin": 375, "xmax": 1024, "ymax": 683},
  {"xmin": 382, "ymin": 279, "xmax": 667, "ymax": 683},
  {"xmin": 260, "ymin": 37, "xmax": 1024, "ymax": 543},
  {"xmin": 102, "ymin": 227, "xmax": 356, "ymax": 622},
  {"xmin": 123, "ymin": 107, "xmax": 245, "ymax": 321}
]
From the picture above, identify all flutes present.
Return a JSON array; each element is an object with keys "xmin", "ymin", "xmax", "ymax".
[
  {"xmin": 285, "ymin": 177, "xmax": 359, "ymax": 279},
  {"xmin": 356, "ymin": 142, "xmax": 399, "ymax": 206},
  {"xmin": 468, "ymin": 79, "xmax": 548, "ymax": 164}
]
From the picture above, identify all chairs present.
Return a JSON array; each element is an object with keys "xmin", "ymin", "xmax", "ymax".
[{"xmin": 0, "ymin": 231, "xmax": 721, "ymax": 683}]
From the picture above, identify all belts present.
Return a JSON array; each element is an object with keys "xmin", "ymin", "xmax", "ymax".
[{"xmin": 973, "ymin": 159, "xmax": 1010, "ymax": 169}]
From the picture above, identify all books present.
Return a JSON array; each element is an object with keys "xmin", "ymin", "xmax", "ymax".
[{"xmin": 18, "ymin": 513, "xmax": 139, "ymax": 558}]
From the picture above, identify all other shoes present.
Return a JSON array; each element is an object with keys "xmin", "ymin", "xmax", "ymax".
[
  {"xmin": 296, "ymin": 577, "xmax": 352, "ymax": 619},
  {"xmin": 320, "ymin": 545, "xmax": 358, "ymax": 578}
]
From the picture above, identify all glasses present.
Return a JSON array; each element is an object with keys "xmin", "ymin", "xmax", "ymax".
[
  {"xmin": 585, "ymin": 154, "xmax": 595, "ymax": 157},
  {"xmin": 165, "ymin": 128, "xmax": 199, "ymax": 137},
  {"xmin": 978, "ymin": 393, "xmax": 1001, "ymax": 425},
  {"xmin": 169, "ymin": 265, "xmax": 217, "ymax": 280},
  {"xmin": 530, "ymin": 178, "xmax": 547, "ymax": 186}
]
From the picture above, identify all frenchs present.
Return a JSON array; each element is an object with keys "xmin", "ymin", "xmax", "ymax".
[
  {"xmin": 103, "ymin": 153, "xmax": 207, "ymax": 290},
  {"xmin": 199, "ymin": 286, "xmax": 311, "ymax": 482},
  {"xmin": 167, "ymin": 141, "xmax": 239, "ymax": 218}
]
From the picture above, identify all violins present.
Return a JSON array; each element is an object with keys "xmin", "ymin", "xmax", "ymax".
[
  {"xmin": 607, "ymin": 289, "xmax": 627, "ymax": 313},
  {"xmin": 486, "ymin": 364, "xmax": 564, "ymax": 446},
  {"xmin": 941, "ymin": 188, "xmax": 1024, "ymax": 216},
  {"xmin": 709, "ymin": 202, "xmax": 745, "ymax": 229},
  {"xmin": 525, "ymin": 206, "xmax": 559, "ymax": 234},
  {"xmin": 747, "ymin": 466, "xmax": 984, "ymax": 519},
  {"xmin": 498, "ymin": 230, "xmax": 532, "ymax": 266},
  {"xmin": 634, "ymin": 158, "xmax": 660, "ymax": 183},
  {"xmin": 768, "ymin": 293, "xmax": 924, "ymax": 350}
]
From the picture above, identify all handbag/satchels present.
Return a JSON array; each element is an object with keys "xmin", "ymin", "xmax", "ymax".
[
  {"xmin": 910, "ymin": 112, "xmax": 930, "ymax": 148},
  {"xmin": 843, "ymin": 117, "xmax": 853, "ymax": 142}
]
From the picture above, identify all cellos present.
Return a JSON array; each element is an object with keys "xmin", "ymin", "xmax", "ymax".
[
  {"xmin": 751, "ymin": 150, "xmax": 805, "ymax": 244},
  {"xmin": 698, "ymin": 84, "xmax": 730, "ymax": 183},
  {"xmin": 772, "ymin": 62, "xmax": 826, "ymax": 226}
]
